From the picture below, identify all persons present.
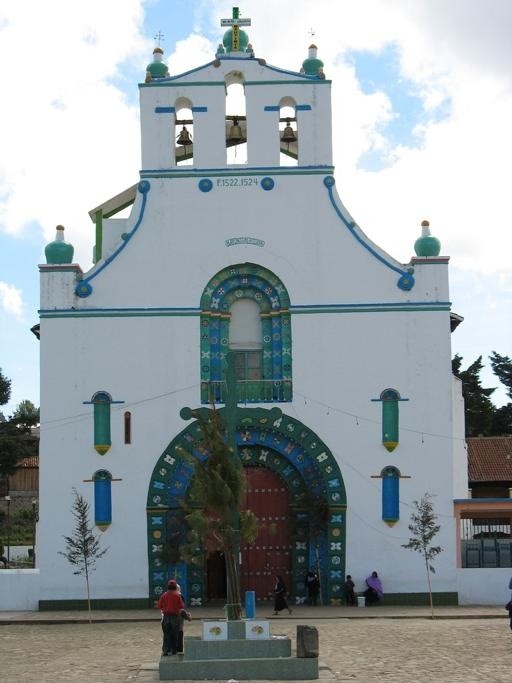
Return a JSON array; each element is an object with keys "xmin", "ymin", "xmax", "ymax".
[
  {"xmin": 365, "ymin": 571, "xmax": 383, "ymax": 606},
  {"xmin": 305, "ymin": 572, "xmax": 320, "ymax": 605},
  {"xmin": 158, "ymin": 579, "xmax": 192, "ymax": 656},
  {"xmin": 272, "ymin": 575, "xmax": 293, "ymax": 615},
  {"xmin": 342, "ymin": 575, "xmax": 355, "ymax": 606}
]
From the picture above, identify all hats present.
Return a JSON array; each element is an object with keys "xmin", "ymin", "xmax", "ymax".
[{"xmin": 168, "ymin": 579, "xmax": 180, "ymax": 591}]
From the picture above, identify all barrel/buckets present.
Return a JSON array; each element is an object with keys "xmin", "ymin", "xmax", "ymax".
[{"xmin": 357, "ymin": 597, "xmax": 366, "ymax": 608}]
